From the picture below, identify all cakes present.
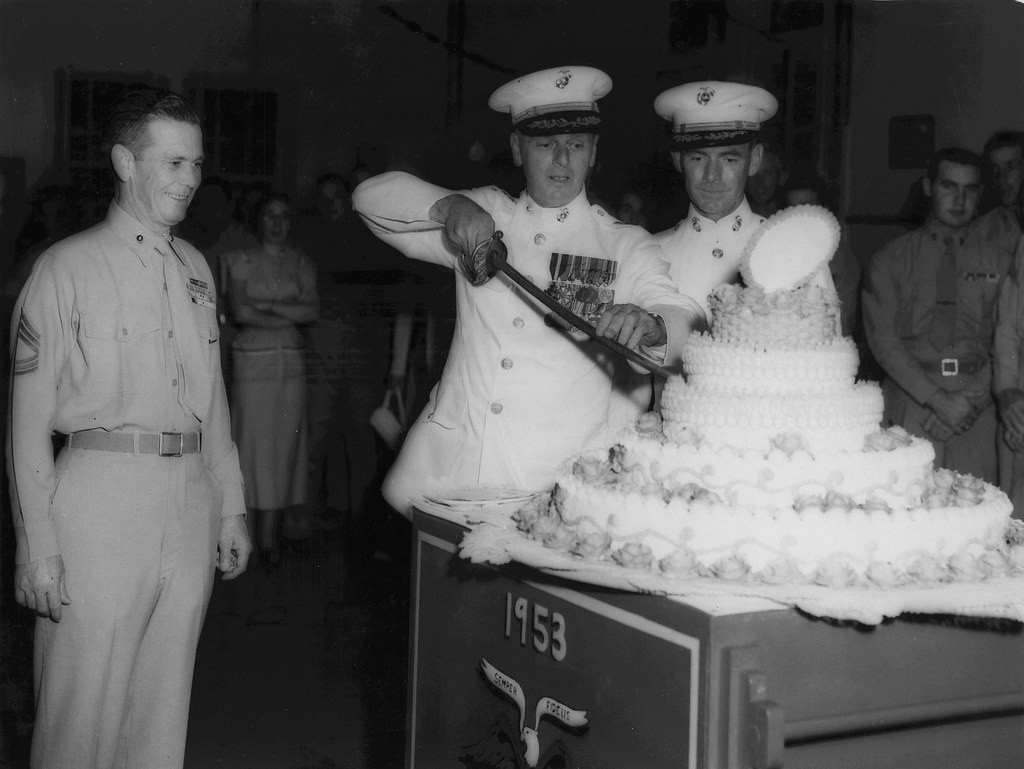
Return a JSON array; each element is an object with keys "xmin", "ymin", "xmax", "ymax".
[{"xmin": 510, "ymin": 203, "xmax": 1024, "ymax": 590}]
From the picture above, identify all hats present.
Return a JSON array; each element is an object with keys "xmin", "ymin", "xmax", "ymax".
[
  {"xmin": 654, "ymin": 82, "xmax": 780, "ymax": 151},
  {"xmin": 487, "ymin": 66, "xmax": 614, "ymax": 137}
]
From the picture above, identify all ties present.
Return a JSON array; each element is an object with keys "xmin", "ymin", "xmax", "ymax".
[
  {"xmin": 156, "ymin": 240, "xmax": 212, "ymax": 423},
  {"xmin": 929, "ymin": 237, "xmax": 958, "ymax": 351}
]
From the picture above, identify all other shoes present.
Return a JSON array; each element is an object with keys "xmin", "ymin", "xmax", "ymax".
[{"xmin": 257, "ymin": 534, "xmax": 310, "ymax": 566}]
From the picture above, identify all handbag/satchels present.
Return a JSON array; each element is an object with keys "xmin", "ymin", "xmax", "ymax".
[{"xmin": 370, "ymin": 385, "xmax": 406, "ymax": 450}]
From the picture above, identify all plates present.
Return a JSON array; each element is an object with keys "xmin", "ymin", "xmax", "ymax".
[{"xmin": 425, "ymin": 492, "xmax": 536, "ymax": 507}]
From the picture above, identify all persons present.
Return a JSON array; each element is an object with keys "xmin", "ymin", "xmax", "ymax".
[
  {"xmin": 214, "ymin": 65, "xmax": 675, "ymax": 570},
  {"xmin": 646, "ymin": 80, "xmax": 1024, "ymax": 533},
  {"xmin": 6, "ymin": 88, "xmax": 251, "ymax": 769}
]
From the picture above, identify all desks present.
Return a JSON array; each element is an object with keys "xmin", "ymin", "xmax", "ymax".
[{"xmin": 411, "ymin": 498, "xmax": 1024, "ymax": 769}]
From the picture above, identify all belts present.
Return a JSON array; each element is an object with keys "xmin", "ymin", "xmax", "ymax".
[
  {"xmin": 921, "ymin": 359, "xmax": 987, "ymax": 378},
  {"xmin": 65, "ymin": 431, "xmax": 201, "ymax": 458}
]
